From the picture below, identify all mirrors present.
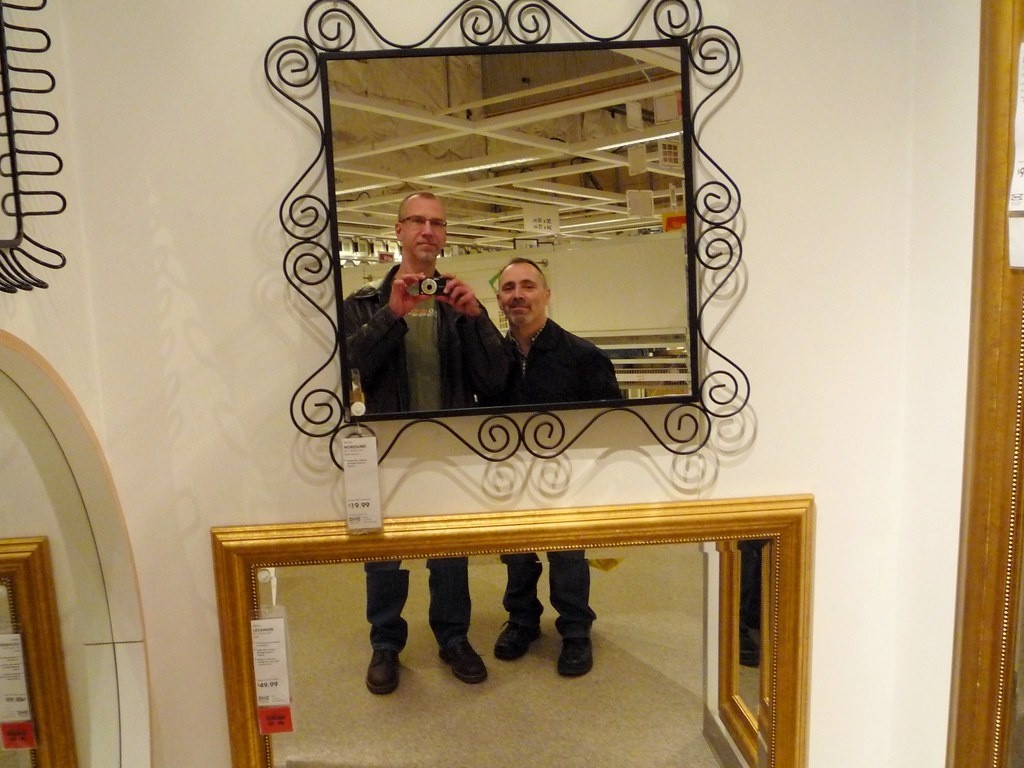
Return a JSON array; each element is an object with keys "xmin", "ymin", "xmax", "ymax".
[
  {"xmin": 265, "ymin": 0, "xmax": 752, "ymax": 464},
  {"xmin": 0, "ymin": 535, "xmax": 77, "ymax": 768},
  {"xmin": 210, "ymin": 493, "xmax": 818, "ymax": 768}
]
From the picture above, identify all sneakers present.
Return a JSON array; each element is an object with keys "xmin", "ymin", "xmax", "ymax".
[
  {"xmin": 494, "ymin": 620, "xmax": 541, "ymax": 658},
  {"xmin": 557, "ymin": 638, "xmax": 591, "ymax": 675}
]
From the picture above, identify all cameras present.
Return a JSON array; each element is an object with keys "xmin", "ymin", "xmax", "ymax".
[{"xmin": 419, "ymin": 277, "xmax": 447, "ymax": 295}]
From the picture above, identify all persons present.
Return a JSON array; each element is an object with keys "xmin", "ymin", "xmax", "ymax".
[
  {"xmin": 738, "ymin": 541, "xmax": 759, "ymax": 667},
  {"xmin": 340, "ymin": 192, "xmax": 510, "ymax": 695},
  {"xmin": 479, "ymin": 258, "xmax": 621, "ymax": 678}
]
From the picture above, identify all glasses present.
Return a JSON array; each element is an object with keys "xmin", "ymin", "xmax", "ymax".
[{"xmin": 398, "ymin": 215, "xmax": 447, "ymax": 229}]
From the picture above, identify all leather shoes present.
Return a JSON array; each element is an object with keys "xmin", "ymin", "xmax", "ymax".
[
  {"xmin": 365, "ymin": 649, "xmax": 400, "ymax": 694},
  {"xmin": 439, "ymin": 641, "xmax": 486, "ymax": 682}
]
